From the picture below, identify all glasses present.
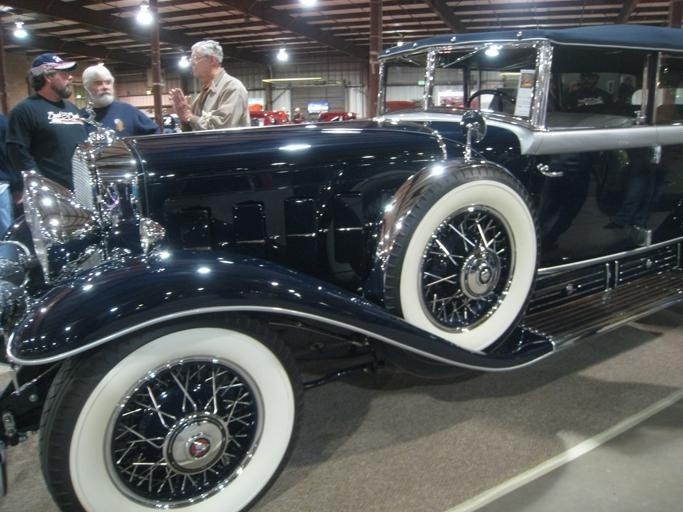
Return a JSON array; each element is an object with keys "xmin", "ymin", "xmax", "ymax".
[{"xmin": 188, "ymin": 55, "xmax": 208, "ymax": 63}]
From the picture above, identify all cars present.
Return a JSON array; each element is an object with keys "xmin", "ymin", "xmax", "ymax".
[{"xmin": 1, "ymin": 26, "xmax": 683, "ymax": 508}]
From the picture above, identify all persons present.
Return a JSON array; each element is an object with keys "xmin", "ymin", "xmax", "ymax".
[
  {"xmin": 527, "ymin": 146, "xmax": 666, "ymax": 250},
  {"xmin": 3, "ymin": 53, "xmax": 94, "ymax": 212},
  {"xmin": 78, "ymin": 65, "xmax": 161, "ymax": 148},
  {"xmin": 167, "ymin": 40, "xmax": 250, "ymax": 136},
  {"xmin": 562, "ymin": 70, "xmax": 615, "ymax": 111},
  {"xmin": 0, "ymin": 113, "xmax": 23, "ymax": 239},
  {"xmin": 292, "ymin": 106, "xmax": 304, "ymax": 121}
]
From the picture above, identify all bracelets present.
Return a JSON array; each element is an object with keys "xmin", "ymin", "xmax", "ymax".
[{"xmin": 184, "ymin": 115, "xmax": 194, "ymax": 123}]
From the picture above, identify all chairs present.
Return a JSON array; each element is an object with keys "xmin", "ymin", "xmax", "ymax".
[
  {"xmin": 655, "ymin": 104, "xmax": 683, "ymax": 127},
  {"xmin": 488, "ymin": 87, "xmax": 519, "ymax": 118}
]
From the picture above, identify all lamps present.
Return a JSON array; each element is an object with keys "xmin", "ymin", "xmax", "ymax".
[
  {"xmin": 13, "ymin": 18, "xmax": 27, "ymax": 39},
  {"xmin": 136, "ymin": 1, "xmax": 153, "ymax": 26},
  {"xmin": 178, "ymin": 49, "xmax": 191, "ymax": 70}
]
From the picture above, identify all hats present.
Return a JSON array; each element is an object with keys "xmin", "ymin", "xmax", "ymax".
[{"xmin": 27, "ymin": 55, "xmax": 80, "ymax": 77}]
